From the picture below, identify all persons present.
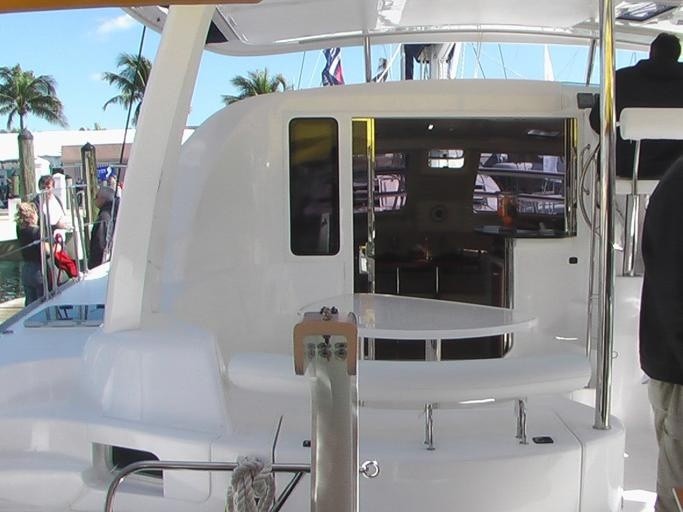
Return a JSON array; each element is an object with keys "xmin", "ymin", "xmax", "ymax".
[
  {"xmin": 108, "ymin": 173, "xmax": 121, "ymax": 197},
  {"xmin": 372, "ymin": 57, "xmax": 390, "ymax": 82},
  {"xmin": 87, "ymin": 186, "xmax": 121, "ymax": 270},
  {"xmin": 31, "ymin": 175, "xmax": 70, "ymax": 283},
  {"xmin": 637, "ymin": 154, "xmax": 683, "ymax": 512},
  {"xmin": 76, "ymin": 177, "xmax": 83, "ymax": 205},
  {"xmin": 588, "ymin": 32, "xmax": 682, "ymax": 178},
  {"xmin": 14, "ymin": 201, "xmax": 63, "ymax": 307}
]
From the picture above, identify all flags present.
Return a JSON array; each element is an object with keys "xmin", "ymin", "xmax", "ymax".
[{"xmin": 320, "ymin": 48, "xmax": 346, "ymax": 86}]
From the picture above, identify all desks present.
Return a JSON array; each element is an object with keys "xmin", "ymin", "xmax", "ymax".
[{"xmin": 295, "ymin": 293, "xmax": 535, "ymax": 361}]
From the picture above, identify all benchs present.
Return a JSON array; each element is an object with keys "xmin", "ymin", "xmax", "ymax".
[{"xmin": 223, "ymin": 341, "xmax": 591, "ymax": 452}]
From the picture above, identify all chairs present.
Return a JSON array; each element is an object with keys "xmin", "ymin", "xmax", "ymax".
[{"xmin": 376, "ymin": 230, "xmax": 506, "ymax": 296}]
